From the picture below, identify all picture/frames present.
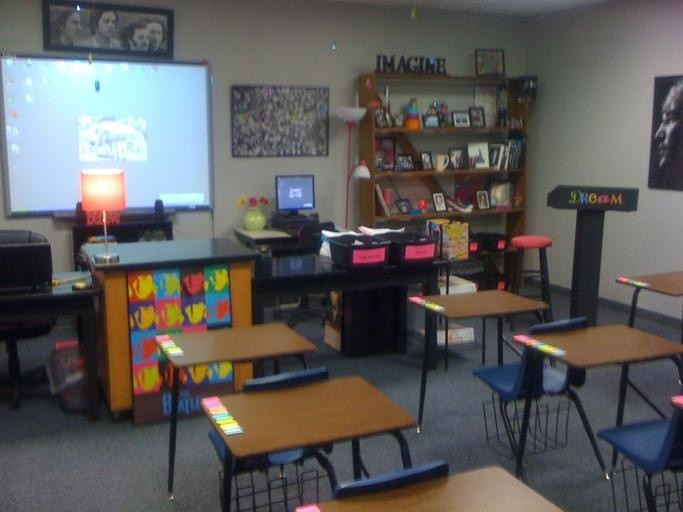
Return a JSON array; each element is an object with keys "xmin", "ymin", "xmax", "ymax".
[
  {"xmin": 422, "ymin": 114, "xmax": 440, "ymax": 128},
  {"xmin": 451, "ymin": 111, "xmax": 470, "ymax": 127},
  {"xmin": 420, "ymin": 151, "xmax": 433, "ymax": 170},
  {"xmin": 432, "ymin": 192, "xmax": 446, "ymax": 212},
  {"xmin": 448, "ymin": 148, "xmax": 465, "ymax": 170},
  {"xmin": 489, "ymin": 144, "xmax": 504, "ymax": 170},
  {"xmin": 468, "ymin": 107, "xmax": 485, "ymax": 128},
  {"xmin": 475, "ymin": 49, "xmax": 506, "ymax": 76},
  {"xmin": 397, "ymin": 153, "xmax": 416, "ymax": 171},
  {"xmin": 476, "ymin": 190, "xmax": 490, "ymax": 209},
  {"xmin": 487, "ymin": 179, "xmax": 513, "ymax": 209},
  {"xmin": 42, "ymin": 0, "xmax": 173, "ymax": 58},
  {"xmin": 395, "ymin": 199, "xmax": 413, "ymax": 214}
]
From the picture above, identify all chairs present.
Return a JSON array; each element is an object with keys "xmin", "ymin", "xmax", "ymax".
[
  {"xmin": 208, "ymin": 368, "xmax": 337, "ymax": 512},
  {"xmin": 596, "ymin": 395, "xmax": 683, "ymax": 512},
  {"xmin": 0, "ymin": 228, "xmax": 53, "ymax": 409},
  {"xmin": 471, "ymin": 316, "xmax": 610, "ymax": 483},
  {"xmin": 273, "ymin": 221, "xmax": 336, "ymax": 328},
  {"xmin": 332, "ymin": 463, "xmax": 450, "ymax": 497}
]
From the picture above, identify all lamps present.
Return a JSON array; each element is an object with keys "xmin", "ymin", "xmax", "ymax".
[
  {"xmin": 80, "ymin": 170, "xmax": 127, "ymax": 265},
  {"xmin": 336, "ymin": 107, "xmax": 371, "ymax": 229}
]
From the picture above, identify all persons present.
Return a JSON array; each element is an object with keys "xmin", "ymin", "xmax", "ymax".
[
  {"xmin": 84, "ymin": 9, "xmax": 121, "ymax": 48},
  {"xmin": 143, "ymin": 17, "xmax": 166, "ymax": 55},
  {"xmin": 50, "ymin": 8, "xmax": 82, "ymax": 47},
  {"xmin": 656, "ymin": 82, "xmax": 683, "ymax": 188},
  {"xmin": 120, "ymin": 20, "xmax": 150, "ymax": 50}
]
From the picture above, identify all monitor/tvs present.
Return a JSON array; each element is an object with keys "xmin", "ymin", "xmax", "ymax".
[{"xmin": 274, "ymin": 174, "xmax": 317, "ymax": 219}]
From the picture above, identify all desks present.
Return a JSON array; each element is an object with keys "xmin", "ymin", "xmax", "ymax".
[
  {"xmin": 0, "ymin": 271, "xmax": 102, "ymax": 422},
  {"xmin": 615, "ymin": 270, "xmax": 683, "ymax": 327},
  {"xmin": 512, "ymin": 323, "xmax": 683, "ymax": 482},
  {"xmin": 406, "ymin": 289, "xmax": 550, "ymax": 429},
  {"xmin": 248, "ymin": 252, "xmax": 450, "ymax": 368},
  {"xmin": 155, "ymin": 321, "xmax": 317, "ymax": 502},
  {"xmin": 234, "ymin": 225, "xmax": 338, "ymax": 258},
  {"xmin": 201, "ymin": 373, "xmax": 418, "ymax": 511}
]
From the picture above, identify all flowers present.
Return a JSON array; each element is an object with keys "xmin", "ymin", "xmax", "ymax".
[{"xmin": 235, "ymin": 197, "xmax": 272, "ymax": 209}]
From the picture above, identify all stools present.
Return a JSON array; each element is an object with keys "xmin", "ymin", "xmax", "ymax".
[{"xmin": 505, "ymin": 235, "xmax": 554, "ymax": 332}]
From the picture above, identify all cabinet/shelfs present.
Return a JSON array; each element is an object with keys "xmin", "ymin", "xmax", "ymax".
[{"xmin": 355, "ymin": 74, "xmax": 529, "ymax": 289}]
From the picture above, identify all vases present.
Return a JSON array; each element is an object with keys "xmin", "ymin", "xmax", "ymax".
[{"xmin": 243, "ymin": 209, "xmax": 265, "ymax": 230}]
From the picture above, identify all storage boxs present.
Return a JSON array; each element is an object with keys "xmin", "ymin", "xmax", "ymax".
[
  {"xmin": 426, "ymin": 219, "xmax": 469, "ymax": 262},
  {"xmin": 469, "ymin": 231, "xmax": 482, "ymax": 254},
  {"xmin": 473, "ymin": 272, "xmax": 510, "ymax": 291},
  {"xmin": 327, "ymin": 235, "xmax": 392, "ymax": 268},
  {"xmin": 477, "ymin": 233, "xmax": 510, "ymax": 253},
  {"xmin": 383, "ymin": 232, "xmax": 438, "ymax": 266}
]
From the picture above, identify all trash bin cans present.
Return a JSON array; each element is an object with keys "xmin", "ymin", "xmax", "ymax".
[{"xmin": 46, "ymin": 346, "xmax": 99, "ymax": 413}]
